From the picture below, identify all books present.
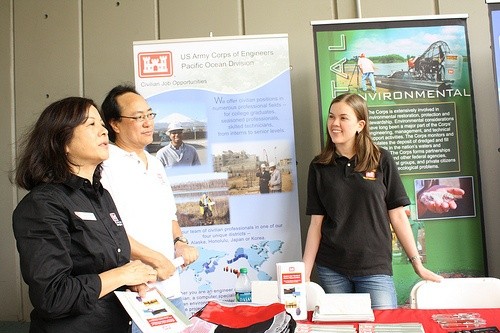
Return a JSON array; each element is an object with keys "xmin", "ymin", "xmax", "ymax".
[
  {"xmin": 113, "ymin": 285, "xmax": 193, "ymax": 333},
  {"xmin": 312, "ymin": 292, "xmax": 373, "ymax": 322}
]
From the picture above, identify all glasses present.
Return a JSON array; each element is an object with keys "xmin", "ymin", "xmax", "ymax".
[{"xmin": 120, "ymin": 112, "xmax": 156, "ymax": 124}]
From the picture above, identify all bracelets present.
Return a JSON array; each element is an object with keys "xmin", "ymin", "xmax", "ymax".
[{"xmin": 408, "ymin": 256, "xmax": 421, "ymax": 264}]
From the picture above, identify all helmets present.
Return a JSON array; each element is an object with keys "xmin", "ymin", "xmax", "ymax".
[{"xmin": 165, "ymin": 123, "xmax": 184, "ymax": 136}]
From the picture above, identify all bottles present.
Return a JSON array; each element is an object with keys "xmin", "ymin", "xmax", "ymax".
[{"xmin": 235, "ymin": 268, "xmax": 252, "ymax": 302}]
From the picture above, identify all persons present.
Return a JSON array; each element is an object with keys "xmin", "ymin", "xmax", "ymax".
[
  {"xmin": 415, "ymin": 177, "xmax": 466, "ymax": 220},
  {"xmin": 438, "ymin": 82, "xmax": 448, "ymax": 95},
  {"xmin": 198, "ymin": 193, "xmax": 220, "ymax": 226},
  {"xmin": 357, "ymin": 54, "xmax": 377, "ymax": 92},
  {"xmin": 10, "ymin": 97, "xmax": 157, "ymax": 333},
  {"xmin": 268, "ymin": 160, "xmax": 283, "ymax": 192},
  {"xmin": 155, "ymin": 123, "xmax": 200, "ymax": 168},
  {"xmin": 100, "ymin": 85, "xmax": 199, "ymax": 281},
  {"xmin": 256, "ymin": 164, "xmax": 271, "ymax": 194},
  {"xmin": 303, "ymin": 93, "xmax": 446, "ymax": 284},
  {"xmin": 408, "ymin": 56, "xmax": 416, "ymax": 72}
]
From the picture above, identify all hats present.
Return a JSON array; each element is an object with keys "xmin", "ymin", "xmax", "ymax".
[
  {"xmin": 260, "ymin": 163, "xmax": 267, "ymax": 169},
  {"xmin": 268, "ymin": 163, "xmax": 276, "ymax": 169}
]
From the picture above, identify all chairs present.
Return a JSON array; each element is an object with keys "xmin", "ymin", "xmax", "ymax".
[{"xmin": 409, "ymin": 277, "xmax": 500, "ymax": 309}]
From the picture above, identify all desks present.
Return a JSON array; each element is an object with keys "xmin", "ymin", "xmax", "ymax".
[{"xmin": 188, "ymin": 306, "xmax": 500, "ymax": 333}]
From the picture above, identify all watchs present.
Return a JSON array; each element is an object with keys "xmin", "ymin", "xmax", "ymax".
[{"xmin": 172, "ymin": 235, "xmax": 189, "ymax": 248}]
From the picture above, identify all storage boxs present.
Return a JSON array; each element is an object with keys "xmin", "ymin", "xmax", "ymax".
[{"xmin": 276, "ymin": 261, "xmax": 308, "ymax": 320}]
